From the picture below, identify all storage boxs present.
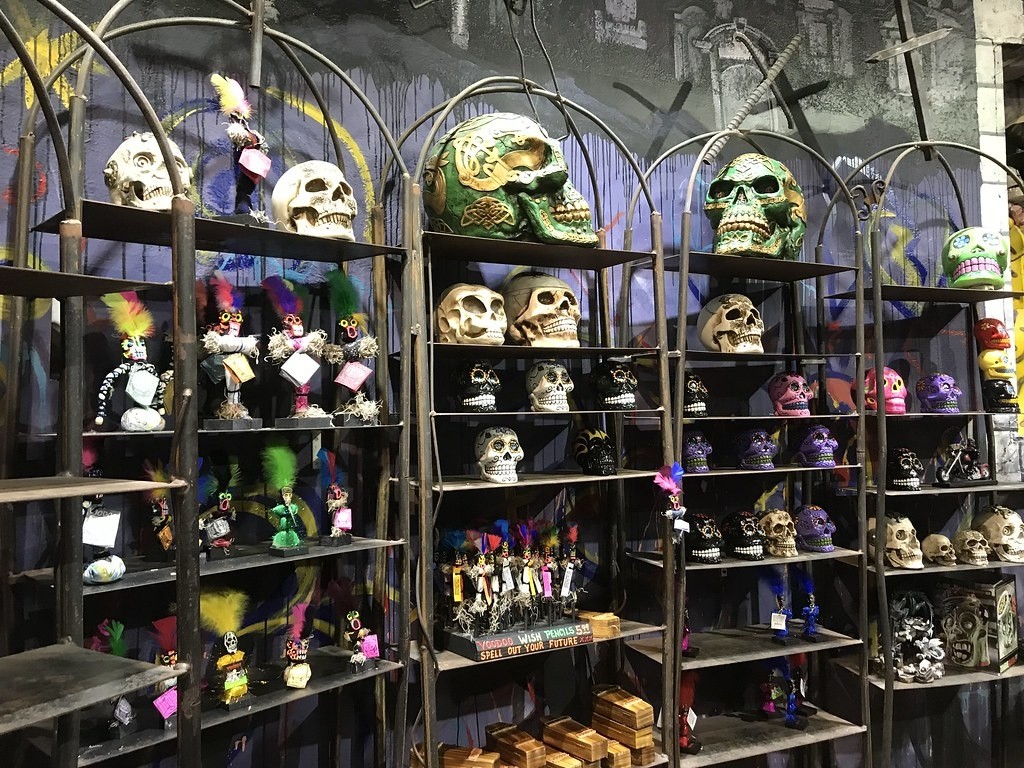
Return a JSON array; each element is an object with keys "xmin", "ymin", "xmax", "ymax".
[{"xmin": 928, "ymin": 570, "xmax": 1019, "ymax": 677}]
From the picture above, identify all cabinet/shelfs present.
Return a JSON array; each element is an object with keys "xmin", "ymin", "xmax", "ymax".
[{"xmin": 0, "ymin": 1, "xmax": 1024, "ymax": 768}]
